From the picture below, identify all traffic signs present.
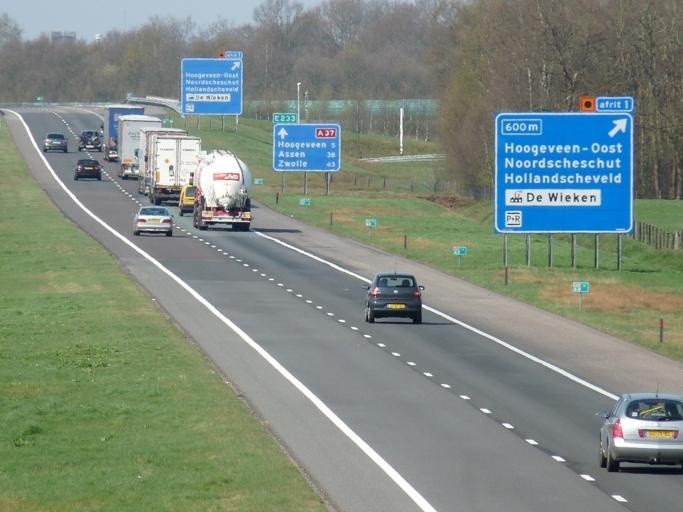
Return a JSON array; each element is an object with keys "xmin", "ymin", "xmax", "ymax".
[
  {"xmin": 272, "ymin": 123, "xmax": 340, "ymax": 173},
  {"xmin": 180, "ymin": 56, "xmax": 241, "ymax": 116},
  {"xmin": 493, "ymin": 110, "xmax": 634, "ymax": 235}
]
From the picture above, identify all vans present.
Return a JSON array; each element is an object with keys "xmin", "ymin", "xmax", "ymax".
[{"xmin": 177, "ymin": 184, "xmax": 195, "ymax": 215}]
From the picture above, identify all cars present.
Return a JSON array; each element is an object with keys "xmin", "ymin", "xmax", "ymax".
[
  {"xmin": 72, "ymin": 159, "xmax": 103, "ymax": 181},
  {"xmin": 98, "ymin": 123, "xmax": 103, "ymax": 138},
  {"xmin": 103, "ymin": 143, "xmax": 117, "ymax": 162},
  {"xmin": 41, "ymin": 133, "xmax": 68, "ymax": 153},
  {"xmin": 594, "ymin": 392, "xmax": 682, "ymax": 472},
  {"xmin": 361, "ymin": 272, "xmax": 425, "ymax": 323},
  {"xmin": 129, "ymin": 206, "xmax": 174, "ymax": 237},
  {"xmin": 76, "ymin": 131, "xmax": 103, "ymax": 151}
]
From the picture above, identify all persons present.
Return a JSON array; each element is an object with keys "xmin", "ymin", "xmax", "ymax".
[
  {"xmin": 627, "ymin": 402, "xmax": 641, "ymax": 416},
  {"xmin": 380, "ymin": 278, "xmax": 388, "ymax": 288},
  {"xmin": 402, "ymin": 279, "xmax": 409, "ymax": 286},
  {"xmin": 665, "ymin": 402, "xmax": 679, "ymax": 416}
]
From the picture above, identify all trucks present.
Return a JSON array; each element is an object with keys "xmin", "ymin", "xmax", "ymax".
[
  {"xmin": 146, "ymin": 135, "xmax": 200, "ymax": 207},
  {"xmin": 137, "ymin": 127, "xmax": 188, "ymax": 196},
  {"xmin": 103, "ymin": 105, "xmax": 144, "ymax": 150},
  {"xmin": 117, "ymin": 115, "xmax": 161, "ymax": 181}
]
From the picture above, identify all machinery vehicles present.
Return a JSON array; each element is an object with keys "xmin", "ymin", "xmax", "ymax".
[{"xmin": 194, "ymin": 149, "xmax": 252, "ymax": 232}]
